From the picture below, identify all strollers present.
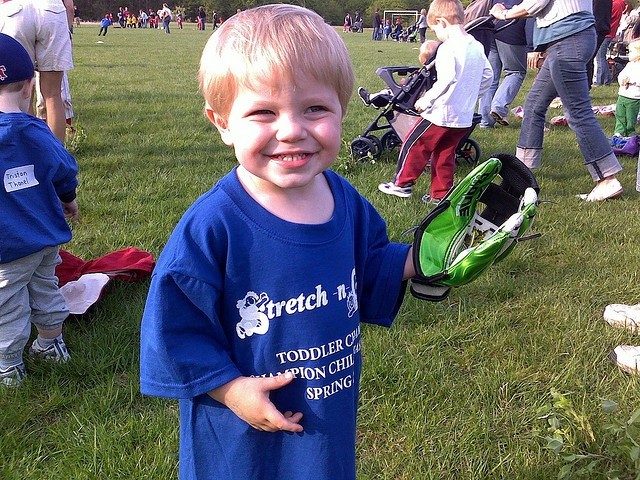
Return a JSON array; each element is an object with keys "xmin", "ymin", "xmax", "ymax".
[
  {"xmin": 347, "ymin": 14, "xmax": 484, "ymax": 165},
  {"xmin": 394, "ymin": 21, "xmax": 422, "ymax": 43}
]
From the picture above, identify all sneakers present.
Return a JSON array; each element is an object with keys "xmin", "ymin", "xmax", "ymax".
[
  {"xmin": 422, "ymin": 194, "xmax": 442, "ymax": 205},
  {"xmin": 602, "ymin": 303, "xmax": 640, "ymax": 331},
  {"xmin": 371, "ymin": 103, "xmax": 380, "ymax": 110},
  {"xmin": 611, "ymin": 343, "xmax": 640, "ymax": 374},
  {"xmin": 0, "ymin": 361, "xmax": 26, "ymax": 386},
  {"xmin": 480, "ymin": 124, "xmax": 498, "ymax": 129},
  {"xmin": 358, "ymin": 87, "xmax": 371, "ymax": 107},
  {"xmin": 576, "ymin": 179, "xmax": 622, "ymax": 202},
  {"xmin": 490, "ymin": 110, "xmax": 509, "ymax": 126},
  {"xmin": 378, "ymin": 182, "xmax": 412, "ymax": 197},
  {"xmin": 27, "ymin": 339, "xmax": 71, "ymax": 363}
]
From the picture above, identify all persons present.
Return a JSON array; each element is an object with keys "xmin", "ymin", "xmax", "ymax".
[
  {"xmin": 358, "ymin": 40, "xmax": 440, "ymax": 109},
  {"xmin": 0, "ymin": 31, "xmax": 78, "ymax": 386},
  {"xmin": 0, "ymin": 0, "xmax": 73, "ymax": 143},
  {"xmin": 373, "ymin": 8, "xmax": 402, "ymax": 39},
  {"xmin": 603, "ymin": 295, "xmax": 639, "ymax": 377},
  {"xmin": 495, "ymin": 0, "xmax": 626, "ymax": 201},
  {"xmin": 525, "ymin": 0, "xmax": 548, "ymax": 72},
  {"xmin": 592, "ymin": 0, "xmax": 640, "ymax": 136},
  {"xmin": 475, "ymin": 0, "xmax": 528, "ymax": 126},
  {"xmin": 416, "ymin": 8, "xmax": 428, "ymax": 42},
  {"xmin": 343, "ymin": 11, "xmax": 363, "ymax": 32},
  {"xmin": 379, "ymin": 0, "xmax": 495, "ymax": 205},
  {"xmin": 140, "ymin": 3, "xmax": 415, "ymax": 480},
  {"xmin": 100, "ymin": 4, "xmax": 221, "ymax": 39}
]
